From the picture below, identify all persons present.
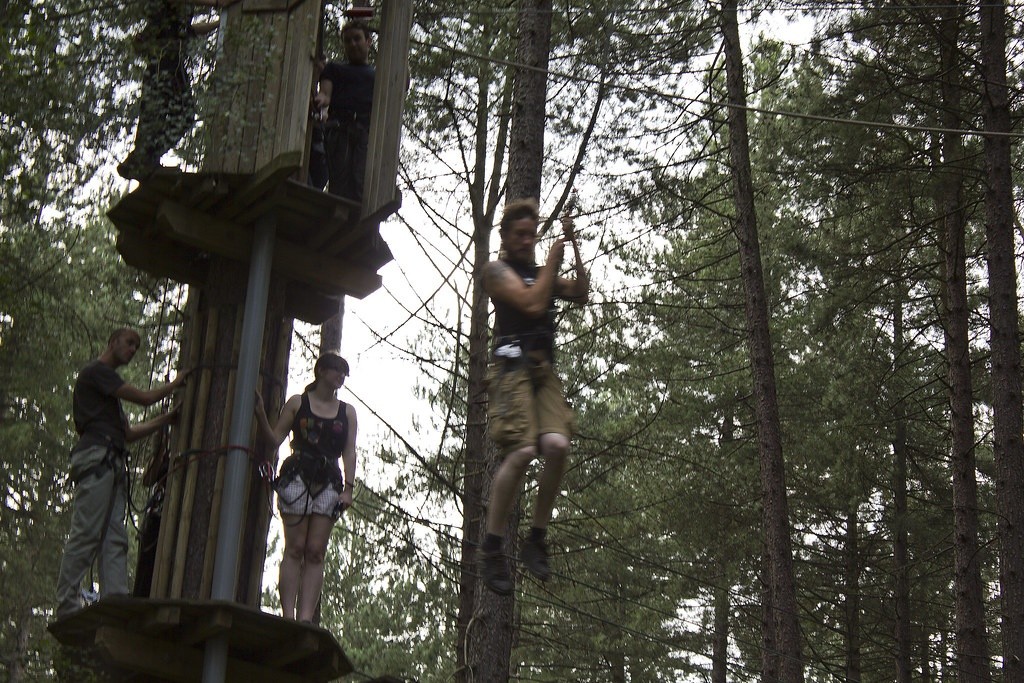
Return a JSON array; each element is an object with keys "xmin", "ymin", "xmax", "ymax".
[
  {"xmin": 308, "ymin": 53, "xmax": 330, "ymax": 191},
  {"xmin": 55, "ymin": 327, "xmax": 194, "ymax": 622},
  {"xmin": 478, "ymin": 197, "xmax": 590, "ymax": 595},
  {"xmin": 117, "ymin": 0, "xmax": 195, "ymax": 182},
  {"xmin": 311, "ymin": 21, "xmax": 410, "ymax": 201},
  {"xmin": 254, "ymin": 352, "xmax": 357, "ymax": 623}
]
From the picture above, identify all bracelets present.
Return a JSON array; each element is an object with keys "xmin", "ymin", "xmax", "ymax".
[{"xmin": 345, "ymin": 479, "xmax": 354, "ymax": 488}]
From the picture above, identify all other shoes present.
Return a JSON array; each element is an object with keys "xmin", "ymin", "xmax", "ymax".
[
  {"xmin": 522, "ymin": 539, "xmax": 550, "ymax": 581},
  {"xmin": 117, "ymin": 158, "xmax": 152, "ymax": 181},
  {"xmin": 479, "ymin": 543, "xmax": 513, "ymax": 595}
]
